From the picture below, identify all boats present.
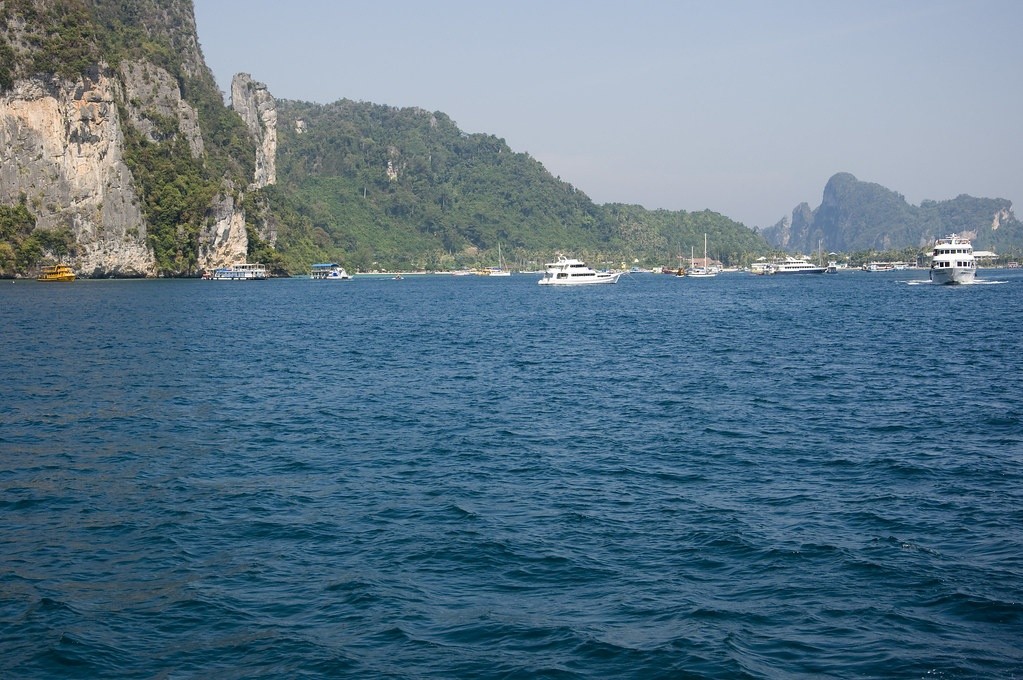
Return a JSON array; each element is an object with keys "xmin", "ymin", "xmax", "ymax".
[
  {"xmin": 628, "ymin": 266, "xmax": 644, "ymax": 273},
  {"xmin": 200, "ymin": 260, "xmax": 279, "ymax": 281},
  {"xmin": 35, "ymin": 262, "xmax": 77, "ymax": 283},
  {"xmin": 750, "ymin": 254, "xmax": 828, "ymax": 275},
  {"xmin": 537, "ymin": 252, "xmax": 625, "ymax": 286},
  {"xmin": 862, "ymin": 261, "xmax": 911, "ymax": 273},
  {"xmin": 928, "ymin": 232, "xmax": 977, "ymax": 285},
  {"xmin": 310, "ymin": 263, "xmax": 355, "ymax": 280},
  {"xmin": 751, "ymin": 263, "xmax": 779, "ymax": 276},
  {"xmin": 826, "ymin": 251, "xmax": 838, "ymax": 274}
]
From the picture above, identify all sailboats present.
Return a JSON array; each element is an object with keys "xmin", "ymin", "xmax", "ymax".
[
  {"xmin": 651, "ymin": 232, "xmax": 725, "ymax": 278},
  {"xmin": 488, "ymin": 242, "xmax": 511, "ymax": 277}
]
